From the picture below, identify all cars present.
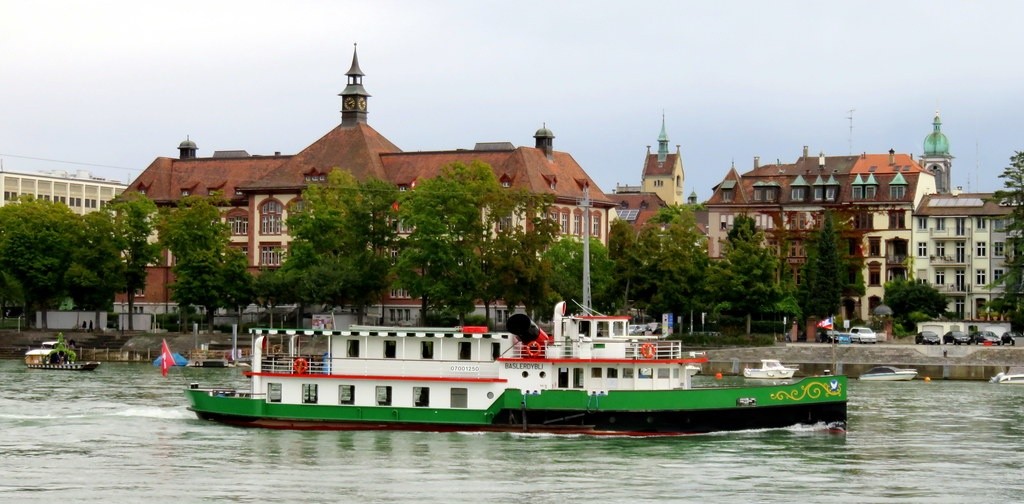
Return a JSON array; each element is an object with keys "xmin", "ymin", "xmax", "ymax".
[
  {"xmin": 943, "ymin": 331, "xmax": 972, "ymax": 345},
  {"xmin": 819, "ymin": 330, "xmax": 840, "ymax": 344},
  {"xmin": 626, "ymin": 323, "xmax": 663, "ymax": 336},
  {"xmin": 971, "ymin": 330, "xmax": 1001, "ymax": 346},
  {"xmin": 915, "ymin": 331, "xmax": 940, "ymax": 345},
  {"xmin": 1001, "ymin": 331, "xmax": 1024, "ymax": 346}
]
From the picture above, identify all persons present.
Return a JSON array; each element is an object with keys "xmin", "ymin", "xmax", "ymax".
[
  {"xmin": 88, "ymin": 320, "xmax": 94, "ymax": 333},
  {"xmin": 82, "ymin": 320, "xmax": 87, "ymax": 333}
]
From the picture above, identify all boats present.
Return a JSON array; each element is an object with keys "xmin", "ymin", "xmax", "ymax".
[
  {"xmin": 685, "ymin": 362, "xmax": 702, "ymax": 376},
  {"xmin": 857, "ymin": 365, "xmax": 917, "ymax": 382},
  {"xmin": 184, "ymin": 356, "xmax": 229, "ymax": 368},
  {"xmin": 24, "ymin": 348, "xmax": 101, "ymax": 372},
  {"xmin": 743, "ymin": 359, "xmax": 800, "ymax": 379},
  {"xmin": 990, "ymin": 371, "xmax": 1024, "ymax": 385},
  {"xmin": 182, "ymin": 184, "xmax": 848, "ymax": 441}
]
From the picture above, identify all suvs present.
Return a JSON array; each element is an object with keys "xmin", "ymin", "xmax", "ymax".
[{"xmin": 849, "ymin": 327, "xmax": 876, "ymax": 344}]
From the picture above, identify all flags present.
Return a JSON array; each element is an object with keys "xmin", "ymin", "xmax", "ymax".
[{"xmin": 161, "ymin": 339, "xmax": 176, "ymax": 377}]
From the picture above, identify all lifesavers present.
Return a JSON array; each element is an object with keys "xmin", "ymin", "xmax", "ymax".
[
  {"xmin": 527, "ymin": 341, "xmax": 541, "ymax": 357},
  {"xmin": 294, "ymin": 358, "xmax": 308, "ymax": 374},
  {"xmin": 641, "ymin": 343, "xmax": 655, "ymax": 358}
]
